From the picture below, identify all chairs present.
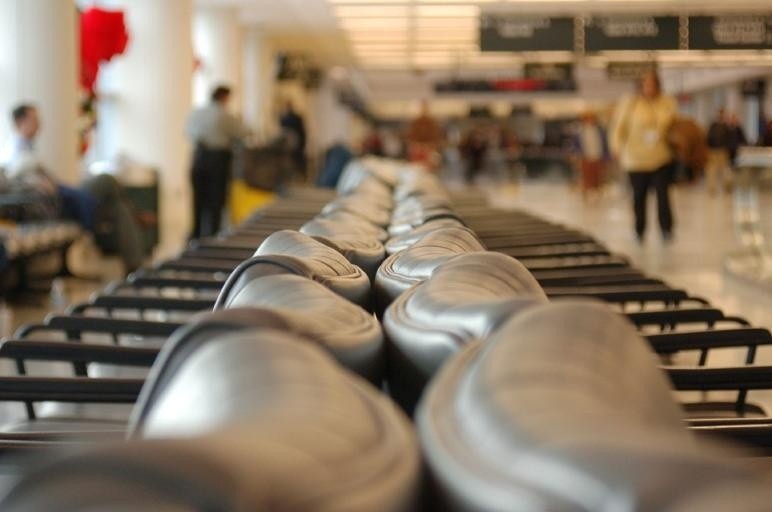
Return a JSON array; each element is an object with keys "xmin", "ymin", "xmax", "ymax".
[{"xmin": 0, "ymin": 155, "xmax": 771, "ymax": 512}]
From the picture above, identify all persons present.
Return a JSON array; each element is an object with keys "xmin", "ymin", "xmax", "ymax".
[
  {"xmin": 464, "ymin": 69, "xmax": 748, "ymax": 244},
  {"xmin": 184, "ymin": 88, "xmax": 245, "ymax": 239},
  {"xmin": 0, "ymin": 106, "xmax": 141, "ymax": 277},
  {"xmin": 280, "ymin": 94, "xmax": 444, "ymax": 187}
]
